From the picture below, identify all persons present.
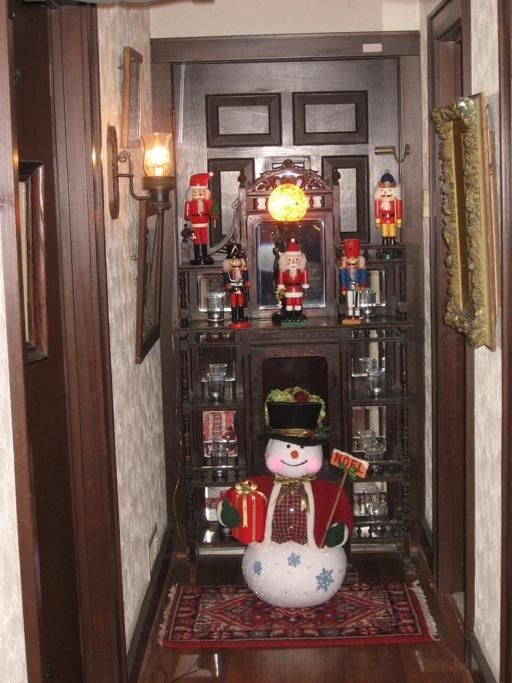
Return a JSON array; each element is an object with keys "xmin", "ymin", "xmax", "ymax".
[
  {"xmin": 374, "ymin": 170, "xmax": 403, "ymax": 251},
  {"xmin": 340, "ymin": 238, "xmax": 368, "ymax": 320},
  {"xmin": 275, "ymin": 239, "xmax": 310, "ymax": 320},
  {"xmin": 184, "ymin": 170, "xmax": 216, "ymax": 264},
  {"xmin": 223, "ymin": 244, "xmax": 252, "ymax": 327}
]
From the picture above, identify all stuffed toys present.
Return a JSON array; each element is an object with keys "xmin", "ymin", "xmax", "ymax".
[{"xmin": 218, "ymin": 401, "xmax": 353, "ymax": 607}]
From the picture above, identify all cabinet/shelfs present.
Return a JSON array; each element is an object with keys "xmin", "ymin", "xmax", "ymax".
[{"xmin": 167, "ymin": 157, "xmax": 425, "ymax": 581}]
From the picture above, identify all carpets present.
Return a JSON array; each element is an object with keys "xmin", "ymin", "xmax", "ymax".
[{"xmin": 156, "ymin": 579, "xmax": 439, "ymax": 649}]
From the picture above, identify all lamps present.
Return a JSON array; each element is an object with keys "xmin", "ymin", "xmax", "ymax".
[
  {"xmin": 267, "ymin": 182, "xmax": 307, "ymax": 221},
  {"xmin": 100, "ymin": 128, "xmax": 177, "ymax": 219}
]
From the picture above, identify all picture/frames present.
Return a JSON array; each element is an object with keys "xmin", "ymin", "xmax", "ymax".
[
  {"xmin": 434, "ymin": 97, "xmax": 498, "ymax": 352},
  {"xmin": 119, "ymin": 46, "xmax": 144, "ymax": 151},
  {"xmin": 138, "ymin": 202, "xmax": 163, "ymax": 361}
]
